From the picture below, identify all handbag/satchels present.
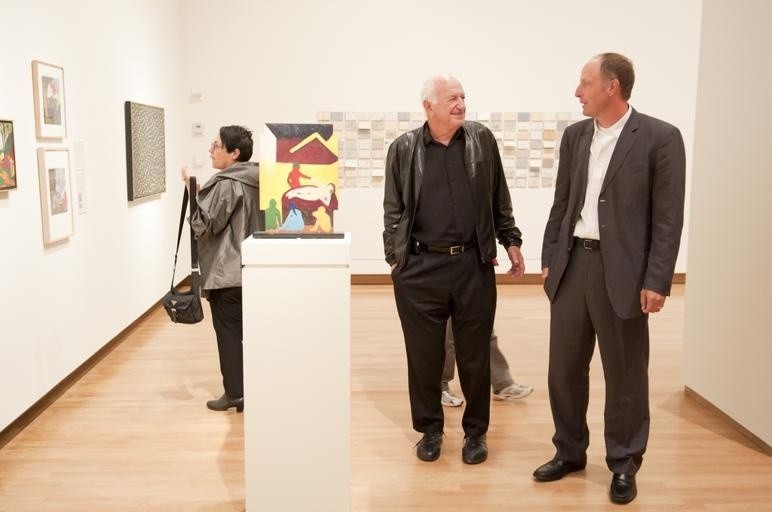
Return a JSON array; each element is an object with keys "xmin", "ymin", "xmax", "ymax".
[{"xmin": 162, "ymin": 288, "xmax": 205, "ymax": 324}]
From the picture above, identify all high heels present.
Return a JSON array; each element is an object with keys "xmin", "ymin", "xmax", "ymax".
[{"xmin": 207, "ymin": 393, "xmax": 245, "ymax": 413}]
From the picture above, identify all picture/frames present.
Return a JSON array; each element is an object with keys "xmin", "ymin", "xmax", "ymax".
[
  {"xmin": 32, "ymin": 59, "xmax": 67, "ymax": 141},
  {"xmin": 1, "ymin": 120, "xmax": 18, "ymax": 191},
  {"xmin": 38, "ymin": 147, "xmax": 74, "ymax": 245}
]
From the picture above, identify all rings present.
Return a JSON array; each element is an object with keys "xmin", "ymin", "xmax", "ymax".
[{"xmin": 656, "ymin": 305, "xmax": 661, "ymax": 311}]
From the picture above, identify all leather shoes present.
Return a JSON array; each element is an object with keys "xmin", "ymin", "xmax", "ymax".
[
  {"xmin": 532, "ymin": 455, "xmax": 587, "ymax": 482},
  {"xmin": 461, "ymin": 433, "xmax": 488, "ymax": 465},
  {"xmin": 610, "ymin": 471, "xmax": 637, "ymax": 505},
  {"xmin": 416, "ymin": 432, "xmax": 443, "ymax": 463}
]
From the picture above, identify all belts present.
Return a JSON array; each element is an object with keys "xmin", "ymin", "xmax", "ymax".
[
  {"xmin": 572, "ymin": 236, "xmax": 601, "ymax": 251},
  {"xmin": 419, "ymin": 241, "xmax": 477, "ymax": 256}
]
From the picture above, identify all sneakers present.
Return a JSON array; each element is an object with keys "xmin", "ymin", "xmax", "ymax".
[
  {"xmin": 440, "ymin": 390, "xmax": 464, "ymax": 408},
  {"xmin": 491, "ymin": 383, "xmax": 534, "ymax": 402}
]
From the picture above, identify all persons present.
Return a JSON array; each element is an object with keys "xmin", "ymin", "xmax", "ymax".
[
  {"xmin": 277, "ymin": 202, "xmax": 305, "ymax": 231},
  {"xmin": 380, "ymin": 70, "xmax": 526, "ymax": 467},
  {"xmin": 529, "ymin": 51, "xmax": 687, "ymax": 503},
  {"xmin": 286, "ymin": 162, "xmax": 312, "ymax": 189},
  {"xmin": 264, "ymin": 199, "xmax": 282, "ymax": 231},
  {"xmin": 440, "ymin": 308, "xmax": 535, "ymax": 408},
  {"xmin": 284, "ymin": 177, "xmax": 335, "ymax": 207},
  {"xmin": 179, "ymin": 124, "xmax": 266, "ymax": 414},
  {"xmin": 309, "ymin": 205, "xmax": 332, "ymax": 233}
]
